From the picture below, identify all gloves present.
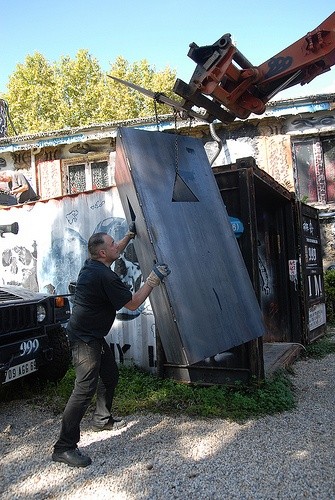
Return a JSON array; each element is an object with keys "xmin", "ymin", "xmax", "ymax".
[
  {"xmin": 146, "ymin": 263, "xmax": 171, "ymax": 287},
  {"xmin": 125, "ymin": 221, "xmax": 136, "ymax": 239}
]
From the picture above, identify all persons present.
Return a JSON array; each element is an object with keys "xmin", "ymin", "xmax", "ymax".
[
  {"xmin": 52, "ymin": 221, "xmax": 171, "ymax": 468},
  {"xmin": 0, "ymin": 170, "xmax": 39, "ymax": 206}
]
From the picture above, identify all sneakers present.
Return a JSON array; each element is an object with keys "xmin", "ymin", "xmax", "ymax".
[
  {"xmin": 92, "ymin": 419, "xmax": 126, "ymax": 432},
  {"xmin": 52, "ymin": 449, "xmax": 92, "ymax": 468}
]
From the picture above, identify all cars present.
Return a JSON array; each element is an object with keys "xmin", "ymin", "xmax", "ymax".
[{"xmin": 1, "ymin": 285, "xmax": 75, "ymax": 397}]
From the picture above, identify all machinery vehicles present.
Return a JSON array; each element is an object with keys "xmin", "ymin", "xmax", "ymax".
[{"xmin": 171, "ymin": 12, "xmax": 335, "ymax": 135}]
